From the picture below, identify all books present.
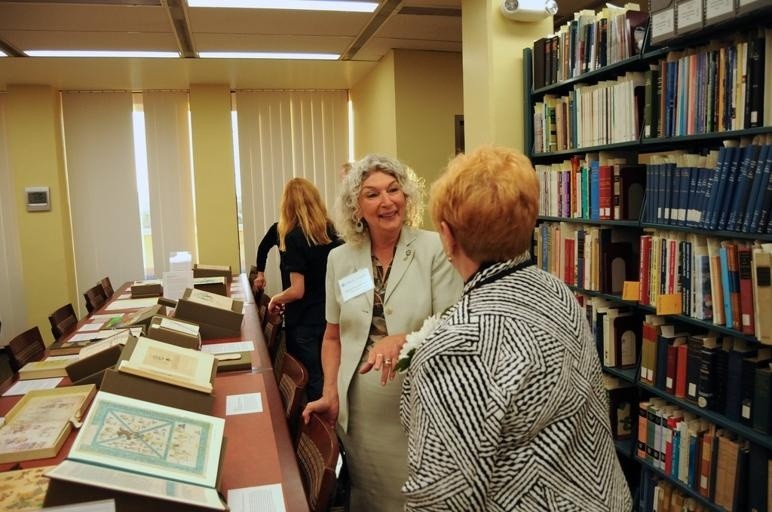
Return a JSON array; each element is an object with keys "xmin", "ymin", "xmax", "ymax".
[{"xmin": 529, "ymin": 0, "xmax": 772, "ymax": 512}]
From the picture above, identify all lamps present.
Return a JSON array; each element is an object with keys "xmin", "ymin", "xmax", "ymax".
[{"xmin": 500, "ymin": 0, "xmax": 559, "ymax": 23}]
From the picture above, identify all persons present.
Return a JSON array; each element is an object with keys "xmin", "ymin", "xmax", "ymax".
[
  {"xmin": 301, "ymin": 153, "xmax": 465, "ymax": 512},
  {"xmin": 266, "ymin": 176, "xmax": 345, "ymax": 398},
  {"xmin": 251, "ymin": 223, "xmax": 307, "ymax": 368},
  {"xmin": 390, "ymin": 142, "xmax": 632, "ymax": 512}
]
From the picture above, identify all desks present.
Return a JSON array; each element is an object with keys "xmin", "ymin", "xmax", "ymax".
[{"xmin": 0, "ymin": 272, "xmax": 312, "ymax": 512}]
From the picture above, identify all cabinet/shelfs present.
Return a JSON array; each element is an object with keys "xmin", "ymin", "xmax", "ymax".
[{"xmin": 522, "ymin": 0, "xmax": 772, "ymax": 511}]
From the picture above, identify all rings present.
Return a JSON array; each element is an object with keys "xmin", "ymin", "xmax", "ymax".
[
  {"xmin": 376, "ymin": 353, "xmax": 383, "ymax": 356},
  {"xmin": 384, "ymin": 358, "xmax": 392, "ymax": 364}
]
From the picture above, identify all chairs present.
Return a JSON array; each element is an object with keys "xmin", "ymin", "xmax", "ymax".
[
  {"xmin": 83, "ymin": 284, "xmax": 107, "ymax": 313},
  {"xmin": 48, "ymin": 304, "xmax": 78, "ymax": 341},
  {"xmin": 97, "ymin": 276, "xmax": 115, "ymax": 300},
  {"xmin": 263, "ymin": 308, "xmax": 283, "ymax": 367},
  {"xmin": 259, "ymin": 293, "xmax": 272, "ymax": 330},
  {"xmin": 248, "ymin": 265, "xmax": 264, "ymax": 308},
  {"xmin": 4, "ymin": 327, "xmax": 46, "ymax": 374},
  {"xmin": 274, "ymin": 352, "xmax": 310, "ymax": 424},
  {"xmin": 294, "ymin": 404, "xmax": 339, "ymax": 512},
  {"xmin": 0, "ymin": 349, "xmax": 16, "ymax": 386}
]
